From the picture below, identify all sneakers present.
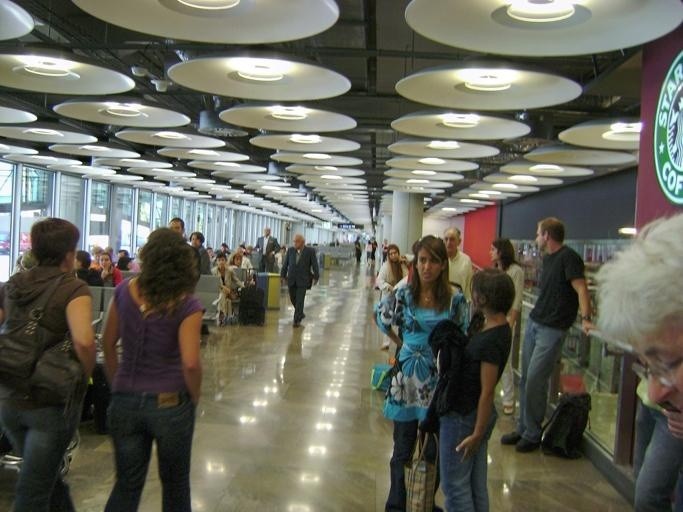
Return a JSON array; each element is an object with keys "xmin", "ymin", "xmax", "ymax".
[{"xmin": 380, "ymin": 345, "xmax": 390, "ymax": 351}]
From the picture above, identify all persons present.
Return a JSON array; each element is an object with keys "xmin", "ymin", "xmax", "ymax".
[
  {"xmin": 96, "ymin": 226, "xmax": 208, "ymax": 512},
  {"xmin": 376, "ymin": 245, "xmax": 409, "ymax": 349},
  {"xmin": 629, "ymin": 365, "xmax": 681, "ymax": 510},
  {"xmin": 74, "ymin": 247, "xmax": 139, "ymax": 287},
  {"xmin": 0, "ymin": 217, "xmax": 97, "ymax": 511},
  {"xmin": 442, "ymin": 227, "xmax": 525, "ymax": 415},
  {"xmin": 372, "ymin": 235, "xmax": 470, "ymax": 511},
  {"xmin": 171, "ymin": 218, "xmax": 285, "ymax": 326},
  {"xmin": 280, "ymin": 234, "xmax": 320, "ymax": 327},
  {"xmin": 588, "ymin": 211, "xmax": 682, "ymax": 414},
  {"xmin": 356, "ymin": 236, "xmax": 389, "ymax": 264},
  {"xmin": 429, "ymin": 270, "xmax": 517, "ymax": 510},
  {"xmin": 501, "ymin": 218, "xmax": 598, "ymax": 453}
]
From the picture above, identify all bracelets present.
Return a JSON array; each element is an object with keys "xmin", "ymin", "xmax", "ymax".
[{"xmin": 582, "ymin": 316, "xmax": 591, "ymax": 320}]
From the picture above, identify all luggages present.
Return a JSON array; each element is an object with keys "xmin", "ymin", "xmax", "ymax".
[{"xmin": 239, "ymin": 282, "xmax": 266, "ymax": 326}]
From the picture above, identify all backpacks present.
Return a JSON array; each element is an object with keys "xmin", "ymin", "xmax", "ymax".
[{"xmin": 540, "ymin": 391, "xmax": 591, "ymax": 456}]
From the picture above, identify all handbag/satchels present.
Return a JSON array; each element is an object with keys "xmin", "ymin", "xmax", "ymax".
[
  {"xmin": 0, "ymin": 318, "xmax": 83, "ymax": 410},
  {"xmin": 370, "ymin": 363, "xmax": 393, "ymax": 392},
  {"xmin": 402, "ymin": 456, "xmax": 437, "ymax": 512}
]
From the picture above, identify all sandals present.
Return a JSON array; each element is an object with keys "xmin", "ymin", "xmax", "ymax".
[{"xmin": 503, "ymin": 398, "xmax": 517, "ymax": 415}]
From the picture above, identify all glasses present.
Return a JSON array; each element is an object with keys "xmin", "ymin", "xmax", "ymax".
[{"xmin": 629, "ymin": 354, "xmax": 683, "ymax": 382}]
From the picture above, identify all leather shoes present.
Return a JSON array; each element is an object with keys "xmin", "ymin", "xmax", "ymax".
[
  {"xmin": 293, "ymin": 312, "xmax": 305, "ymax": 327},
  {"xmin": 502, "ymin": 430, "xmax": 539, "ymax": 452}
]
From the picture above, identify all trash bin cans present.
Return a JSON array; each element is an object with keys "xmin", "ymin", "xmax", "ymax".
[
  {"xmin": 256, "ymin": 272, "xmax": 282, "ymax": 310},
  {"xmin": 319, "ymin": 251, "xmax": 331, "ymax": 270}
]
